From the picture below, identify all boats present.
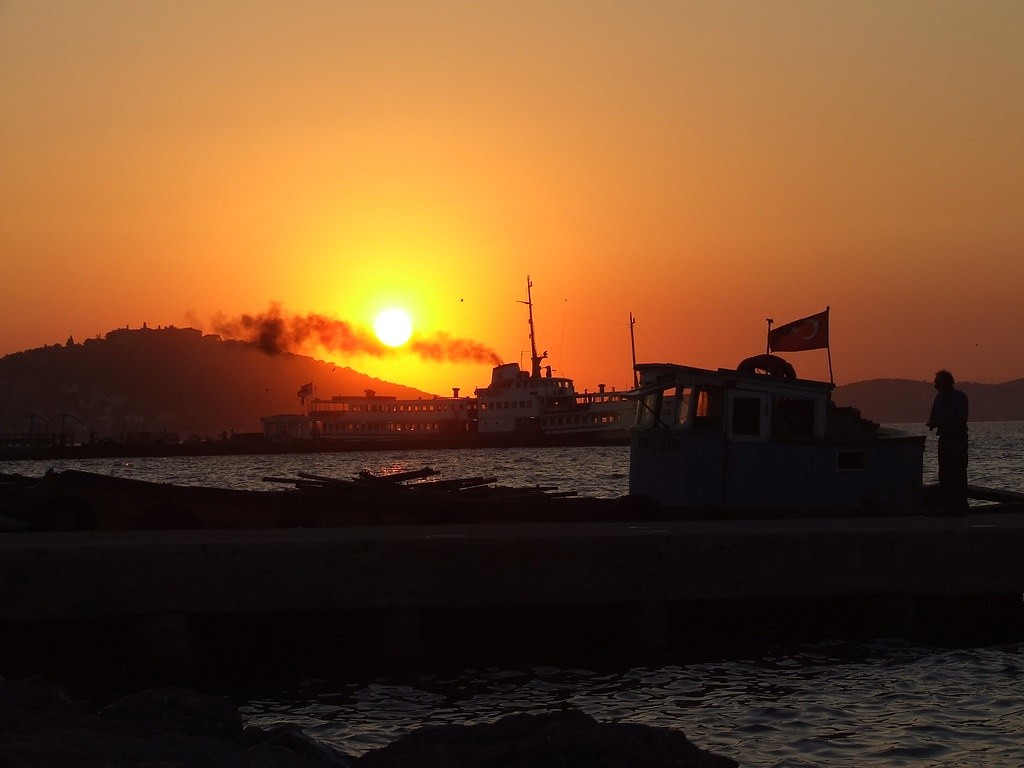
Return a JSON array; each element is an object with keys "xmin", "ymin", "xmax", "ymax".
[
  {"xmin": 297, "ymin": 275, "xmax": 691, "ymax": 449},
  {"xmin": 620, "ymin": 305, "xmax": 926, "ymax": 518}
]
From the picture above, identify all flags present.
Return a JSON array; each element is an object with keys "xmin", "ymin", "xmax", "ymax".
[{"xmin": 768, "ymin": 306, "xmax": 829, "ymax": 352}]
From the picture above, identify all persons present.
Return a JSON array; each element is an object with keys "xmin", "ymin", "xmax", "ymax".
[{"xmin": 926, "ymin": 369, "xmax": 970, "ymax": 516}]
